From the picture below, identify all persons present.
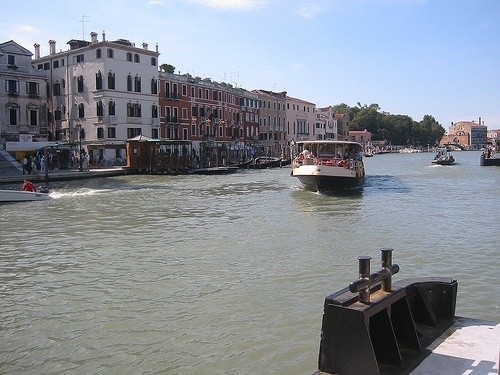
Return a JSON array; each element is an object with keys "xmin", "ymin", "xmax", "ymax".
[
  {"xmin": 20, "ymin": 130, "xmax": 101, "ymax": 175},
  {"xmin": 434, "ymin": 151, "xmax": 445, "ymax": 160},
  {"xmin": 21, "ymin": 178, "xmax": 37, "ymax": 192},
  {"xmin": 193, "ymin": 148, "xmax": 260, "ymax": 165},
  {"xmin": 296, "ymin": 147, "xmax": 353, "ymax": 166},
  {"xmin": 480, "ymin": 141, "xmax": 496, "ymax": 158}
]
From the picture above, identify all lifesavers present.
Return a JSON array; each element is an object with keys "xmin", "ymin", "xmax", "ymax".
[{"xmin": 336, "ymin": 160, "xmax": 350, "ymax": 170}]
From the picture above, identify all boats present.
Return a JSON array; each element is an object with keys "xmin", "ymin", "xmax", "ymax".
[
  {"xmin": 0, "ymin": 186, "xmax": 50, "ymax": 202},
  {"xmin": 431, "ymin": 147, "xmax": 455, "ymax": 165},
  {"xmin": 480, "ymin": 151, "xmax": 500, "ymax": 166},
  {"xmin": 289, "ymin": 140, "xmax": 365, "ymax": 191},
  {"xmin": 365, "ymin": 153, "xmax": 373, "ymax": 157},
  {"xmin": 192, "ymin": 157, "xmax": 288, "ymax": 174}
]
